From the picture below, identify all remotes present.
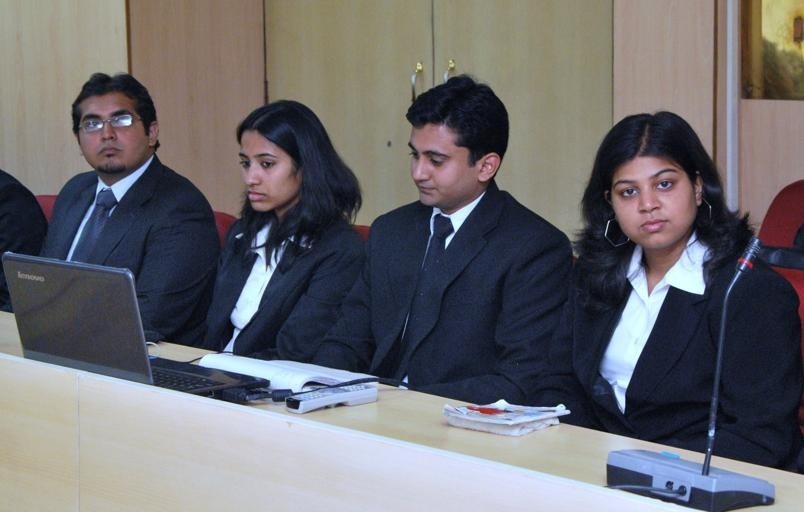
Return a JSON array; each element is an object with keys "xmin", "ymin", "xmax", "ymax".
[{"xmin": 284, "ymin": 383, "xmax": 377, "ymax": 414}]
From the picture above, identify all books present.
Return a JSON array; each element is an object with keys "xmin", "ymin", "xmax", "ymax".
[
  {"xmin": 197, "ymin": 350, "xmax": 381, "ymax": 395},
  {"xmin": 443, "ymin": 398, "xmax": 572, "ymax": 439}
]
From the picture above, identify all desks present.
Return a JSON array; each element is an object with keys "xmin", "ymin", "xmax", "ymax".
[{"xmin": 0, "ymin": 310, "xmax": 803, "ymax": 512}]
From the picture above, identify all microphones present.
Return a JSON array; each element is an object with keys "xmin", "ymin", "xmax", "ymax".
[{"xmin": 607, "ymin": 236, "xmax": 775, "ymax": 511}]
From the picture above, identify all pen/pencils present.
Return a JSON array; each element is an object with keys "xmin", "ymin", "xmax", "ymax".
[{"xmin": 469, "ymin": 405, "xmax": 558, "ymax": 416}]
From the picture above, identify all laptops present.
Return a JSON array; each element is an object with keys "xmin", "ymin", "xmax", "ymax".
[{"xmin": 2, "ymin": 252, "xmax": 270, "ymax": 400}]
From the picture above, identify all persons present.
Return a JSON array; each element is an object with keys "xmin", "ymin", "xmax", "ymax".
[
  {"xmin": 527, "ymin": 110, "xmax": 803, "ymax": 474},
  {"xmin": 314, "ymin": 73, "xmax": 572, "ymax": 404},
  {"xmin": 179, "ymin": 98, "xmax": 366, "ymax": 361},
  {"xmin": 1, "ymin": 168, "xmax": 49, "ymax": 314},
  {"xmin": 4, "ymin": 72, "xmax": 220, "ymax": 348}
]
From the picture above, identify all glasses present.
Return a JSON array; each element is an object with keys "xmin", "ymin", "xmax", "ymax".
[{"xmin": 77, "ymin": 113, "xmax": 141, "ymax": 133}]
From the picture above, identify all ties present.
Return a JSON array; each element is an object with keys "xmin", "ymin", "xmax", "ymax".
[
  {"xmin": 70, "ymin": 191, "xmax": 118, "ymax": 264},
  {"xmin": 391, "ymin": 214, "xmax": 454, "ymax": 381}
]
